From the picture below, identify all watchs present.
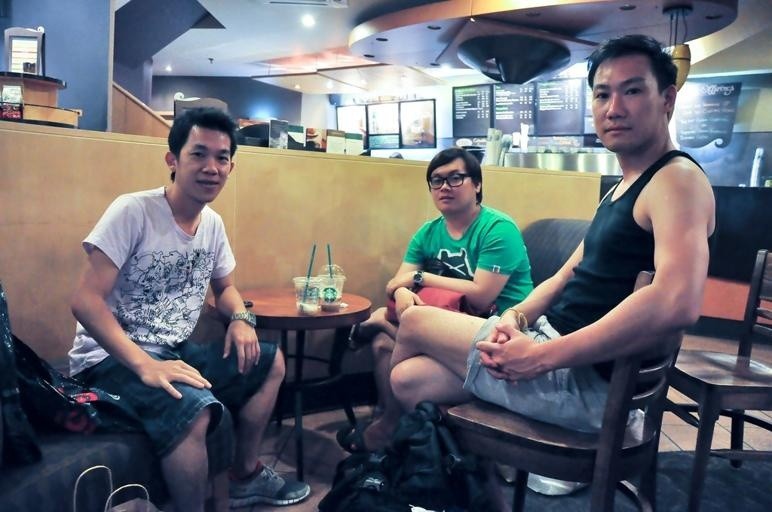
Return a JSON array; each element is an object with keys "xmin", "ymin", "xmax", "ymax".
[
  {"xmin": 411, "ymin": 271, "xmax": 423, "ymax": 293},
  {"xmin": 229, "ymin": 311, "xmax": 257, "ymax": 327}
]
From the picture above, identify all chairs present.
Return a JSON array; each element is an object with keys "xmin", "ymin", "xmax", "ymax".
[
  {"xmin": 655, "ymin": 248, "xmax": 772, "ymax": 512},
  {"xmin": 446, "ymin": 269, "xmax": 683, "ymax": 511},
  {"xmin": 0, "ymin": 355, "xmax": 233, "ymax": 512},
  {"xmin": 520, "ymin": 217, "xmax": 591, "ymax": 289}
]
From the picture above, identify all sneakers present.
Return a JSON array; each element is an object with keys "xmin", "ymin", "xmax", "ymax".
[{"xmin": 227, "ymin": 460, "xmax": 311, "ymax": 509}]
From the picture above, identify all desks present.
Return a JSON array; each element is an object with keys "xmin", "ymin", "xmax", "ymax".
[{"xmin": 202, "ymin": 286, "xmax": 372, "ymax": 485}]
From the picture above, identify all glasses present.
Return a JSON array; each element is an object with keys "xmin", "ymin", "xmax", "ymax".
[{"xmin": 427, "ymin": 172, "xmax": 469, "ymax": 190}]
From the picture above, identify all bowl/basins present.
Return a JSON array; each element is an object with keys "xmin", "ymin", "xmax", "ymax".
[{"xmin": 459, "ymin": 145, "xmax": 484, "ymax": 165}]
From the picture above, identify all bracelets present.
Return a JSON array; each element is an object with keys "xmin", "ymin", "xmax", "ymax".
[{"xmin": 499, "ymin": 307, "xmax": 528, "ymax": 329}]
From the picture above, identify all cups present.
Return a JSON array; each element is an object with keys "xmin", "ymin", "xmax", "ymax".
[
  {"xmin": 292, "ymin": 276, "xmax": 321, "ymax": 318},
  {"xmin": 536, "ymin": 144, "xmax": 592, "ymax": 153},
  {"xmin": 314, "ymin": 264, "xmax": 347, "ymax": 312},
  {"xmin": 484, "ymin": 127, "xmax": 502, "ymax": 166}
]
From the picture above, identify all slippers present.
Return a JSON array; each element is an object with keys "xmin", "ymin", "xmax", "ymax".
[
  {"xmin": 336, "ymin": 423, "xmax": 371, "ymax": 454},
  {"xmin": 345, "ymin": 322, "xmax": 372, "ymax": 353}
]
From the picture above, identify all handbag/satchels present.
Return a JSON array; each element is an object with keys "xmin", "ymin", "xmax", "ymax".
[
  {"xmin": 73, "ymin": 464, "xmax": 159, "ymax": 512},
  {"xmin": 384, "ymin": 285, "xmax": 498, "ymax": 324},
  {"xmin": 317, "ymin": 401, "xmax": 472, "ymax": 511}
]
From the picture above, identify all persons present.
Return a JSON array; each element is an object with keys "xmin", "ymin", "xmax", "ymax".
[
  {"xmin": 336, "ymin": 34, "xmax": 717, "ymax": 455},
  {"xmin": 67, "ymin": 107, "xmax": 311, "ymax": 512},
  {"xmin": 356, "ymin": 147, "xmax": 534, "ymax": 441}
]
